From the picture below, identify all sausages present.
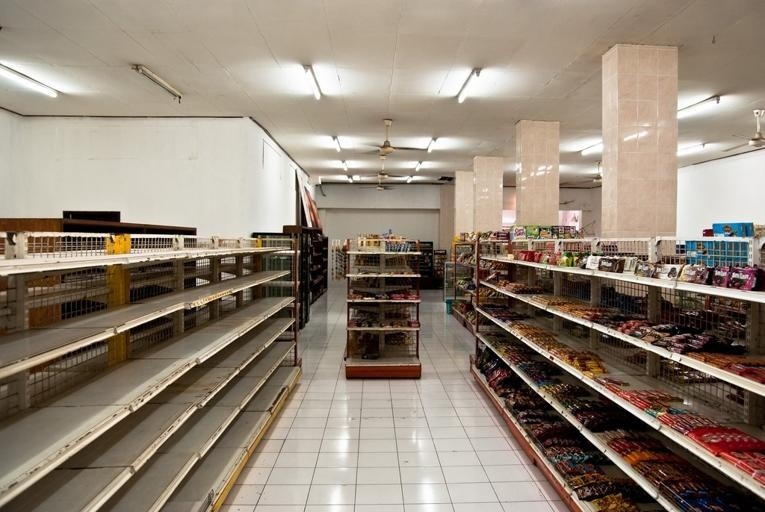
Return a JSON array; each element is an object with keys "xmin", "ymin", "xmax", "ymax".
[
  {"xmin": 594, "ymin": 376, "xmax": 765, "ymax": 487},
  {"xmin": 530, "ymin": 295, "xmax": 618, "ymax": 322},
  {"xmin": 496, "ymin": 279, "xmax": 544, "ymax": 294},
  {"xmin": 687, "ymin": 351, "xmax": 764, "ymax": 383},
  {"xmin": 487, "ymin": 308, "xmax": 607, "ymax": 379}
]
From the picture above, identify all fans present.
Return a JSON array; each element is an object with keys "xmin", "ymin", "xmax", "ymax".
[
  {"xmin": 722, "ymin": 109, "xmax": 765, "ymax": 152},
  {"xmin": 360, "ymin": 156, "xmax": 404, "ymax": 179},
  {"xmin": 359, "ymin": 179, "xmax": 401, "ymax": 192},
  {"xmin": 577, "ymin": 160, "xmax": 602, "ymax": 180},
  {"xmin": 352, "ymin": 119, "xmax": 429, "ymax": 155}
]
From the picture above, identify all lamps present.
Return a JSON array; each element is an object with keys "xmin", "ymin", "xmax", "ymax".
[
  {"xmin": 581, "ymin": 95, "xmax": 720, "ymax": 156},
  {"xmin": 0, "ymin": 64, "xmax": 59, "ymax": 98},
  {"xmin": 407, "ymin": 68, "xmax": 485, "ymax": 184},
  {"xmin": 302, "ymin": 64, "xmax": 354, "ymax": 184},
  {"xmin": 130, "ymin": 65, "xmax": 183, "ymax": 105}
]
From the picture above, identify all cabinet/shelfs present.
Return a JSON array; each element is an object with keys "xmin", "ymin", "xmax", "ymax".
[
  {"xmin": 452, "ymin": 244, "xmax": 765, "ymax": 512},
  {"xmin": 253, "ymin": 225, "xmax": 447, "ymax": 379},
  {"xmin": 1, "ymin": 219, "xmax": 302, "ymax": 512}
]
